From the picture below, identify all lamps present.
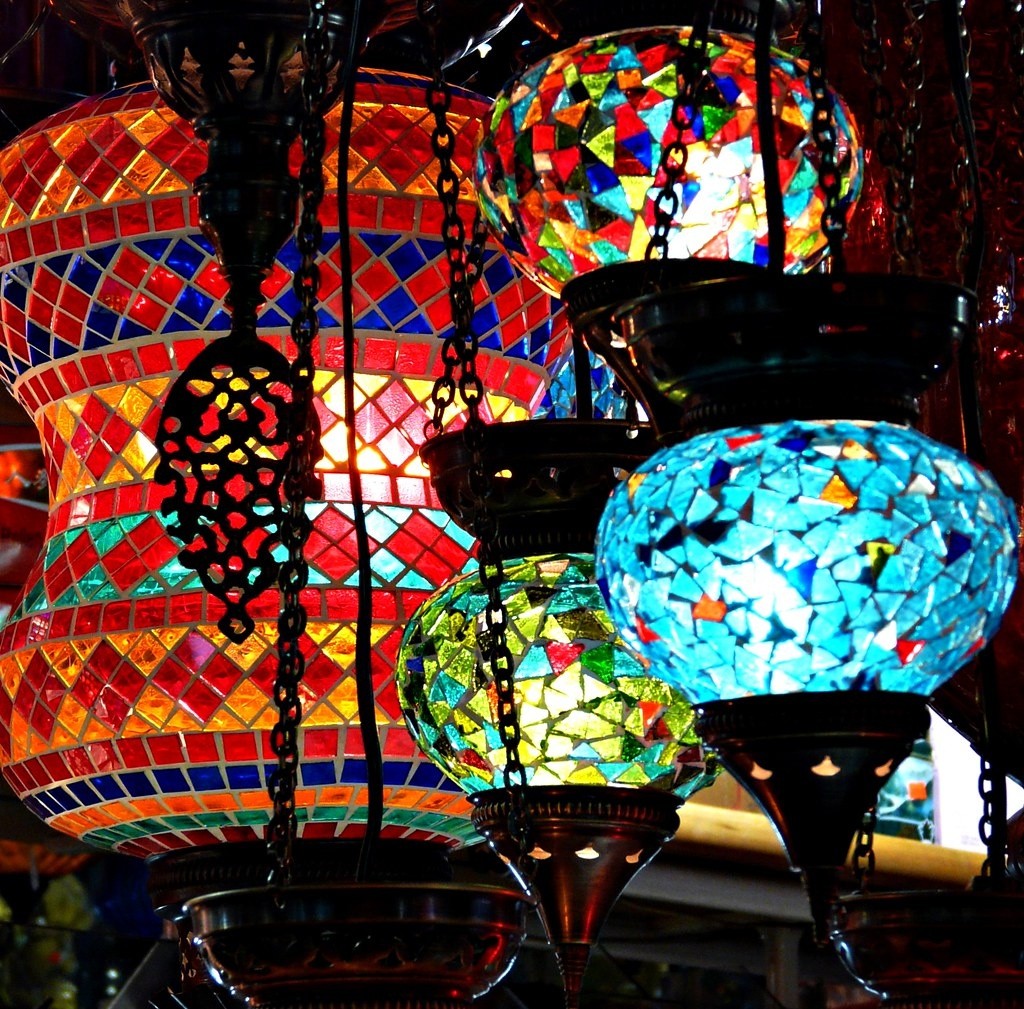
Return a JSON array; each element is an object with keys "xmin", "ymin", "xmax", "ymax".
[
  {"xmin": 595, "ymin": 272, "xmax": 1024, "ymax": 1008},
  {"xmin": 392, "ymin": 421, "xmax": 724, "ymax": 1009}
]
